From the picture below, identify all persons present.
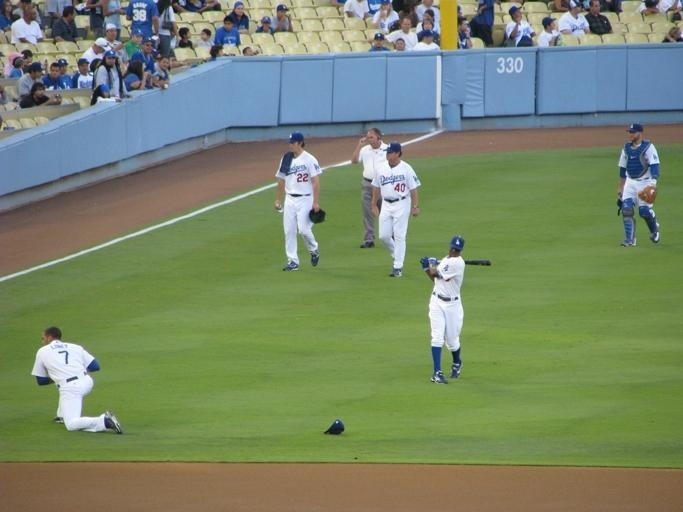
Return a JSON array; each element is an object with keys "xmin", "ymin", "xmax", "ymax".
[
  {"xmin": 370, "ymin": 140, "xmax": 421, "ymax": 277},
  {"xmin": 616, "ymin": 123, "xmax": 661, "ymax": 246},
  {"xmin": 1, "ymin": 1, "xmax": 294, "ymax": 106},
  {"xmin": 420, "ymin": 235, "xmax": 491, "ymax": 385},
  {"xmin": 351, "ymin": 127, "xmax": 394, "ymax": 248},
  {"xmin": 31, "ymin": 327, "xmax": 123, "ymax": 434},
  {"xmin": 274, "ymin": 132, "xmax": 325, "ymax": 272}
]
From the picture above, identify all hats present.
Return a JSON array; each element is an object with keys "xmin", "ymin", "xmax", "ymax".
[
  {"xmin": 235, "ymin": 1, "xmax": 289, "ymax": 24},
  {"xmin": 10, "ymin": 52, "xmax": 68, "ymax": 70},
  {"xmin": 324, "ymin": 420, "xmax": 344, "ymax": 434},
  {"xmin": 569, "ymin": 0, "xmax": 583, "ymax": 9},
  {"xmin": 449, "ymin": 236, "xmax": 465, "ymax": 251},
  {"xmin": 542, "ymin": 17, "xmax": 556, "ymax": 26},
  {"xmin": 421, "ymin": 30, "xmax": 436, "ymax": 36},
  {"xmin": 508, "ymin": 5, "xmax": 522, "ymax": 16},
  {"xmin": 383, "ymin": 143, "xmax": 401, "ymax": 151},
  {"xmin": 374, "ymin": 33, "xmax": 384, "ymax": 42},
  {"xmin": 77, "ymin": 22, "xmax": 156, "ymax": 67},
  {"xmin": 286, "ymin": 132, "xmax": 303, "ymax": 143}
]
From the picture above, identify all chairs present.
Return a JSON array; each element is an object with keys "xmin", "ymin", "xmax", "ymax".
[
  {"xmin": 1, "ymin": 0, "xmax": 199, "ymax": 139},
  {"xmin": 200, "ymin": 0, "xmax": 440, "ymax": 56},
  {"xmin": 458, "ymin": 0, "xmax": 682, "ymax": 49}
]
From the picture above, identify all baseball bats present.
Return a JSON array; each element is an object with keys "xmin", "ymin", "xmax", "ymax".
[{"xmin": 436, "ymin": 261, "xmax": 492, "ymax": 266}]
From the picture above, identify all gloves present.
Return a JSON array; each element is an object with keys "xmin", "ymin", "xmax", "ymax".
[
  {"xmin": 428, "ymin": 257, "xmax": 437, "ymax": 270},
  {"xmin": 421, "ymin": 257, "xmax": 428, "ymax": 270}
]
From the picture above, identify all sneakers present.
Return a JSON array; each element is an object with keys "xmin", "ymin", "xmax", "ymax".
[
  {"xmin": 390, "ymin": 269, "xmax": 401, "ymax": 277},
  {"xmin": 311, "ymin": 250, "xmax": 320, "ymax": 267},
  {"xmin": 652, "ymin": 223, "xmax": 660, "ymax": 243},
  {"xmin": 105, "ymin": 412, "xmax": 121, "ymax": 434},
  {"xmin": 622, "ymin": 238, "xmax": 636, "ymax": 247},
  {"xmin": 283, "ymin": 262, "xmax": 299, "ymax": 271},
  {"xmin": 449, "ymin": 362, "xmax": 462, "ymax": 379},
  {"xmin": 54, "ymin": 416, "xmax": 64, "ymax": 422},
  {"xmin": 430, "ymin": 370, "xmax": 448, "ymax": 384},
  {"xmin": 361, "ymin": 241, "xmax": 375, "ymax": 248}
]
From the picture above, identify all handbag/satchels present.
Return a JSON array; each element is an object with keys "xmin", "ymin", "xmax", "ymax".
[{"xmin": 161, "ymin": 20, "xmax": 173, "ymax": 30}]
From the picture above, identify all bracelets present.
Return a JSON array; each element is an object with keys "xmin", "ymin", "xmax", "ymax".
[{"xmin": 412, "ymin": 204, "xmax": 419, "ymax": 209}]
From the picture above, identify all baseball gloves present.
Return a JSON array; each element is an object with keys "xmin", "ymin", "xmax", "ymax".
[
  {"xmin": 309, "ymin": 208, "xmax": 326, "ymax": 223},
  {"xmin": 639, "ymin": 186, "xmax": 656, "ymax": 202}
]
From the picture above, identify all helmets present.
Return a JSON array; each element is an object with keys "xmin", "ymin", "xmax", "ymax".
[{"xmin": 626, "ymin": 124, "xmax": 642, "ymax": 132}]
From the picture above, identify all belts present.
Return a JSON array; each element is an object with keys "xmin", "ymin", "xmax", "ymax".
[
  {"xmin": 432, "ymin": 292, "xmax": 459, "ymax": 302},
  {"xmin": 290, "ymin": 194, "xmax": 310, "ymax": 196},
  {"xmin": 365, "ymin": 179, "xmax": 373, "ymax": 183},
  {"xmin": 385, "ymin": 195, "xmax": 406, "ymax": 202},
  {"xmin": 57, "ymin": 371, "xmax": 88, "ymax": 388}
]
